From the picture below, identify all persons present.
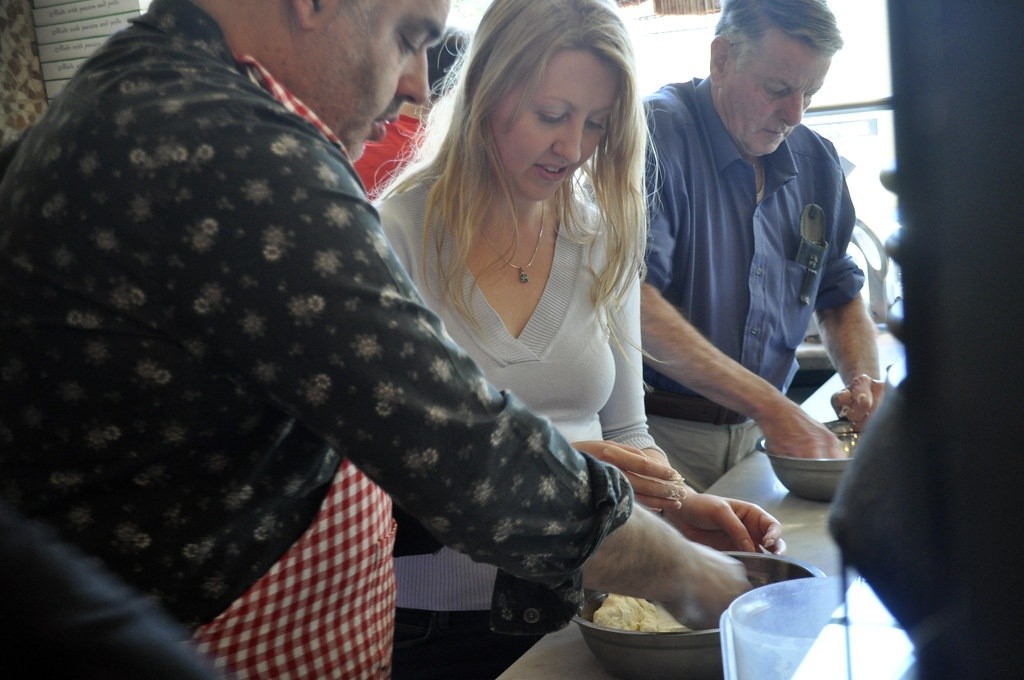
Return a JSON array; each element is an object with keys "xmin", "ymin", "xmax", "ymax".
[
  {"xmin": 375, "ymin": 0, "xmax": 788, "ymax": 680},
  {"xmin": 2, "ymin": 0, "xmax": 752, "ymax": 680},
  {"xmin": 639, "ymin": 0, "xmax": 887, "ymax": 497},
  {"xmin": 352, "ymin": 29, "xmax": 469, "ymax": 198}
]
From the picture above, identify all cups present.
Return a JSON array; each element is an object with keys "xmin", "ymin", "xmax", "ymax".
[{"xmin": 721, "ymin": 576, "xmax": 856, "ymax": 680}]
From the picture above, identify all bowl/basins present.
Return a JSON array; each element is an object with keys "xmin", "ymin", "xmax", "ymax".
[
  {"xmin": 755, "ymin": 421, "xmax": 861, "ymax": 501},
  {"xmin": 572, "ymin": 551, "xmax": 827, "ymax": 680}
]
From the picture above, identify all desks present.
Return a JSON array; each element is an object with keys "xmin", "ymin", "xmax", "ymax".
[{"xmin": 499, "ymin": 320, "xmax": 916, "ymax": 678}]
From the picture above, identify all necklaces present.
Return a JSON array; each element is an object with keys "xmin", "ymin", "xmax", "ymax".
[{"xmin": 475, "ymin": 199, "xmax": 546, "ymax": 282}]
